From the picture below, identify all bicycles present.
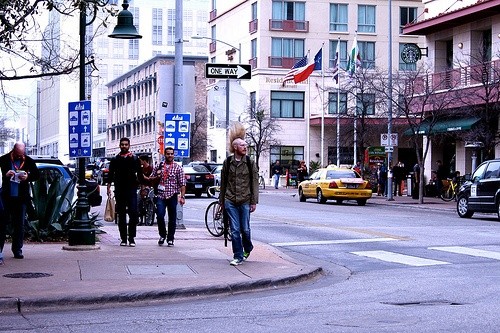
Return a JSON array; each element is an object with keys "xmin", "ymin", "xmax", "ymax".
[
  {"xmin": 204, "ymin": 185, "xmax": 230, "ymax": 247},
  {"xmin": 440, "ymin": 178, "xmax": 461, "ymax": 202}
]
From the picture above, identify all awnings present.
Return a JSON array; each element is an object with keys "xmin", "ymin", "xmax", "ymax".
[
  {"xmin": 405, "ymin": 115, "xmax": 482, "ymax": 135},
  {"xmin": 100, "ymin": 157, "xmax": 115, "ymax": 159},
  {"xmin": 133, "ymin": 152, "xmax": 151, "ymax": 155}
]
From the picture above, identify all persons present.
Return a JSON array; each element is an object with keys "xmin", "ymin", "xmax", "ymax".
[
  {"xmin": 352, "ymin": 160, "xmax": 361, "ymax": 177},
  {"xmin": 204, "ymin": 160, "xmax": 212, "ymax": 172},
  {"xmin": 148, "ymin": 147, "xmax": 186, "ymax": 247},
  {"xmin": 134, "ymin": 155, "xmax": 157, "ymax": 226},
  {"xmin": 272, "ymin": 160, "xmax": 281, "ymax": 189},
  {"xmin": 99, "ymin": 159, "xmax": 103, "ymax": 167},
  {"xmin": 107, "ymin": 137, "xmax": 150, "ymax": 247},
  {"xmin": 297, "ymin": 160, "xmax": 308, "ymax": 188},
  {"xmin": 219, "ymin": 124, "xmax": 259, "ymax": 265},
  {"xmin": 0, "ymin": 141, "xmax": 40, "ymax": 263},
  {"xmin": 373, "ymin": 161, "xmax": 406, "ymax": 197}
]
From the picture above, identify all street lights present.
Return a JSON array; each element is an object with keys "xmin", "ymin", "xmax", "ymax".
[
  {"xmin": 190, "ymin": 36, "xmax": 242, "ymax": 85},
  {"xmin": 67, "ymin": 0, "xmax": 142, "ymax": 245}
]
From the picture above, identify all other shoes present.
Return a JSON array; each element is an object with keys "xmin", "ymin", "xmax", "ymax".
[
  {"xmin": 120, "ymin": 242, "xmax": 127, "ymax": 246},
  {"xmin": 0, "ymin": 253, "xmax": 5, "ymax": 264},
  {"xmin": 145, "ymin": 220, "xmax": 153, "ymax": 226},
  {"xmin": 230, "ymin": 259, "xmax": 243, "ymax": 266},
  {"xmin": 158, "ymin": 237, "xmax": 165, "ymax": 245},
  {"xmin": 168, "ymin": 241, "xmax": 174, "ymax": 247},
  {"xmin": 14, "ymin": 252, "xmax": 24, "ymax": 259},
  {"xmin": 243, "ymin": 252, "xmax": 250, "ymax": 260},
  {"xmin": 129, "ymin": 242, "xmax": 136, "ymax": 246},
  {"xmin": 136, "ymin": 220, "xmax": 139, "ymax": 225}
]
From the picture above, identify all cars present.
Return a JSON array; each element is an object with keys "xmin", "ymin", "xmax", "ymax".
[
  {"xmin": 297, "ymin": 163, "xmax": 373, "ymax": 206},
  {"xmin": 180, "ymin": 162, "xmax": 224, "ymax": 198},
  {"xmin": 68, "ymin": 161, "xmax": 110, "ymax": 186}
]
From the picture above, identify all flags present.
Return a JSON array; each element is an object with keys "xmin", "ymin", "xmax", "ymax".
[
  {"xmin": 332, "ymin": 43, "xmax": 338, "ymax": 84},
  {"xmin": 346, "ymin": 36, "xmax": 361, "ymax": 72},
  {"xmin": 294, "ymin": 48, "xmax": 322, "ymax": 84},
  {"xmin": 283, "ymin": 55, "xmax": 308, "ymax": 87}
]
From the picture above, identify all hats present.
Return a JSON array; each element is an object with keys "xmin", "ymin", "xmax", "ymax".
[{"xmin": 300, "ymin": 160, "xmax": 305, "ymax": 164}]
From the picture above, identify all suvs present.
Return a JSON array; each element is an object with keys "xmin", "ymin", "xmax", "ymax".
[
  {"xmin": 26, "ymin": 154, "xmax": 75, "ymax": 212},
  {"xmin": 456, "ymin": 158, "xmax": 500, "ymax": 220}
]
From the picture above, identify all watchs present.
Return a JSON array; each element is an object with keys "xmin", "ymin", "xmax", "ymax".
[{"xmin": 181, "ymin": 196, "xmax": 185, "ymax": 198}]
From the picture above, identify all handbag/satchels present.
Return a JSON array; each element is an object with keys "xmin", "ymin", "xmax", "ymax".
[
  {"xmin": 153, "ymin": 170, "xmax": 162, "ymax": 195},
  {"xmin": 103, "ymin": 199, "xmax": 115, "ymax": 222}
]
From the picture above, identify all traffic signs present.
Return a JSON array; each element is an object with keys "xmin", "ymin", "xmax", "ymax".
[{"xmin": 204, "ymin": 63, "xmax": 252, "ymax": 80}]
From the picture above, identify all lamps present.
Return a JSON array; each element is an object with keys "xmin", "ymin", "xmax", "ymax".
[{"xmin": 458, "ymin": 42, "xmax": 463, "ymax": 49}]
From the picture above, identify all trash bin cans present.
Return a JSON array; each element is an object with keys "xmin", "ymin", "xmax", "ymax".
[{"xmin": 406, "ymin": 174, "xmax": 415, "ymax": 196}]
